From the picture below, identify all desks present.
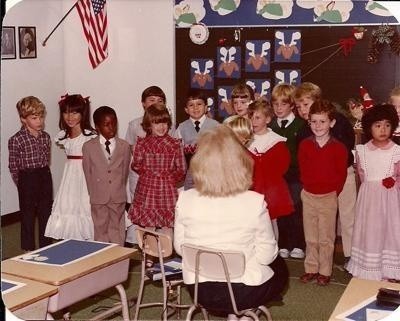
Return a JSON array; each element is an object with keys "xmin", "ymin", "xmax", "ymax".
[
  {"xmin": 1, "ymin": 270, "xmax": 60, "ymax": 321},
  {"xmin": 326, "ymin": 275, "xmax": 399, "ymax": 320},
  {"xmin": 1, "ymin": 236, "xmax": 140, "ymax": 321}
]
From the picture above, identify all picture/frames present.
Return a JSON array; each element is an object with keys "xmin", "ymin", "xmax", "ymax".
[
  {"xmin": 18, "ymin": 24, "xmax": 37, "ymax": 59},
  {"xmin": 0, "ymin": 24, "xmax": 15, "ymax": 61}
]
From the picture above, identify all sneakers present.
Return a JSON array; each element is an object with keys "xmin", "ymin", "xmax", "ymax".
[
  {"xmin": 316, "ymin": 273, "xmax": 330, "ymax": 287},
  {"xmin": 300, "ymin": 272, "xmax": 316, "ymax": 283},
  {"xmin": 144, "ymin": 260, "xmax": 154, "ymax": 270},
  {"xmin": 290, "ymin": 248, "xmax": 304, "ymax": 260},
  {"xmin": 279, "ymin": 249, "xmax": 289, "ymax": 259},
  {"xmin": 225, "ymin": 313, "xmax": 255, "ymax": 321}
]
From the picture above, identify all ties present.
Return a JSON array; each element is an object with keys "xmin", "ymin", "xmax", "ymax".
[
  {"xmin": 105, "ymin": 141, "xmax": 111, "ymax": 155},
  {"xmin": 280, "ymin": 120, "xmax": 288, "ymax": 130},
  {"xmin": 195, "ymin": 121, "xmax": 201, "ymax": 134}
]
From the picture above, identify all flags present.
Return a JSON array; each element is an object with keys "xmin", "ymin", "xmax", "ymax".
[{"xmin": 75, "ymin": 1, "xmax": 108, "ymax": 70}]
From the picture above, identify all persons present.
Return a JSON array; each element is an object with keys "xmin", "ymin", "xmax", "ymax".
[{"xmin": 173, "ymin": 126, "xmax": 289, "ymax": 321}]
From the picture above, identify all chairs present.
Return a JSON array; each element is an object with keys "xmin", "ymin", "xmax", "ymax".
[
  {"xmin": 180, "ymin": 240, "xmax": 272, "ymax": 321},
  {"xmin": 131, "ymin": 223, "xmax": 208, "ymax": 321}
]
